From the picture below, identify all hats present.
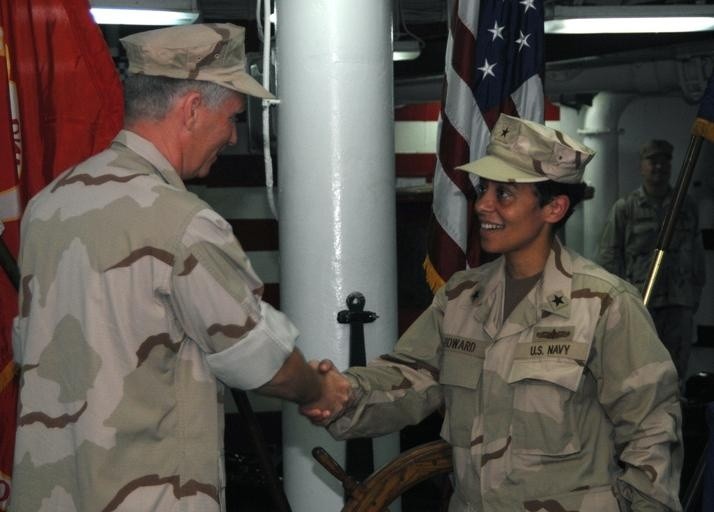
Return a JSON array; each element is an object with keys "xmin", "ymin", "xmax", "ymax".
[
  {"xmin": 637, "ymin": 138, "xmax": 672, "ymax": 160},
  {"xmin": 453, "ymin": 112, "xmax": 596, "ymax": 185},
  {"xmin": 118, "ymin": 23, "xmax": 277, "ymax": 100}
]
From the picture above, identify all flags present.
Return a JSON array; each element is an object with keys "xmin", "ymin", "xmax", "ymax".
[
  {"xmin": 421, "ymin": 1, "xmax": 546, "ymax": 295},
  {"xmin": 183, "ymin": 102, "xmax": 557, "ymax": 439},
  {"xmin": 0, "ymin": 0, "xmax": 125, "ymax": 512}
]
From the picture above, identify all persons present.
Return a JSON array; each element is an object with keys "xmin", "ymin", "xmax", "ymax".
[
  {"xmin": 0, "ymin": 20, "xmax": 353, "ymax": 512},
  {"xmin": 593, "ymin": 139, "xmax": 704, "ymax": 382},
  {"xmin": 295, "ymin": 112, "xmax": 687, "ymax": 511}
]
